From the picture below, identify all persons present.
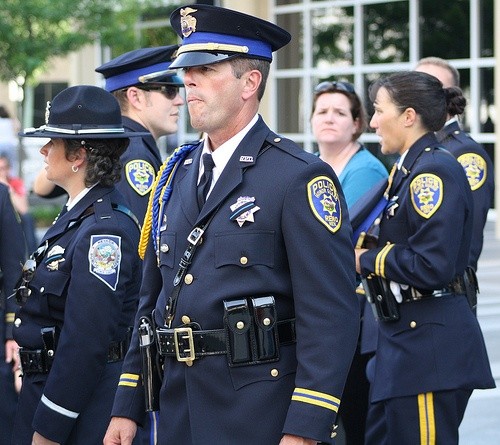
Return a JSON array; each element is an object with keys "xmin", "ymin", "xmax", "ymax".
[
  {"xmin": 0, "ymin": 153, "xmax": 37, "ymax": 254},
  {"xmin": 356, "ymin": 70, "xmax": 497, "ymax": 445},
  {"xmin": 310, "ymin": 82, "xmax": 390, "ymax": 221},
  {"xmin": 12, "ymin": 87, "xmax": 146, "ymax": 445},
  {"xmin": 413, "ymin": 57, "xmax": 493, "ymax": 317},
  {"xmin": 94, "ymin": 43, "xmax": 184, "ymax": 228},
  {"xmin": 104, "ymin": 4, "xmax": 360, "ymax": 445}
]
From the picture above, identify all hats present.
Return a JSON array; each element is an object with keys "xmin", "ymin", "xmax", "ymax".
[
  {"xmin": 94, "ymin": 43, "xmax": 186, "ymax": 93},
  {"xmin": 167, "ymin": 5, "xmax": 293, "ymax": 71},
  {"xmin": 17, "ymin": 85, "xmax": 152, "ymax": 140}
]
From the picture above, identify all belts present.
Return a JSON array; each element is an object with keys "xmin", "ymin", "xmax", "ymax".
[
  {"xmin": 395, "ymin": 269, "xmax": 478, "ymax": 302},
  {"xmin": 155, "ymin": 316, "xmax": 298, "ymax": 362},
  {"xmin": 19, "ymin": 342, "xmax": 121, "ymax": 375}
]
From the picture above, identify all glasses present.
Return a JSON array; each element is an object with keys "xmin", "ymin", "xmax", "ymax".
[
  {"xmin": 122, "ymin": 83, "xmax": 180, "ymax": 100},
  {"xmin": 313, "ymin": 81, "xmax": 356, "ymax": 96}
]
